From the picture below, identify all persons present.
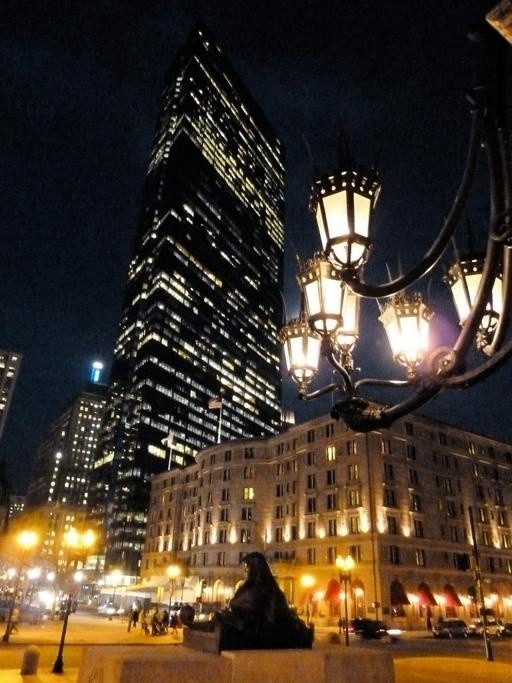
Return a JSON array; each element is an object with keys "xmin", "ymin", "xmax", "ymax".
[
  {"xmin": 8, "ymin": 603, "xmax": 20, "ymax": 635},
  {"xmin": 127, "ymin": 609, "xmax": 179, "ymax": 635},
  {"xmin": 213, "ymin": 552, "xmax": 301, "ymax": 649}
]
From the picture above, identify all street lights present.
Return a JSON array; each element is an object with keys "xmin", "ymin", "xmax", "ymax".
[
  {"xmin": 167, "ymin": 564, "xmax": 179, "ymax": 617},
  {"xmin": 279, "ymin": 87, "xmax": 511, "ymax": 434},
  {"xmin": 2, "ymin": 530, "xmax": 38, "ymax": 643},
  {"xmin": 112, "ymin": 569, "xmax": 121, "ymax": 604},
  {"xmin": 302, "ymin": 575, "xmax": 314, "ymax": 626},
  {"xmin": 51, "ymin": 526, "xmax": 95, "ymax": 674},
  {"xmin": 336, "ymin": 554, "xmax": 355, "ymax": 646}
]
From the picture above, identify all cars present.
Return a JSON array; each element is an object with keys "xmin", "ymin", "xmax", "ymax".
[
  {"xmin": 350, "ymin": 619, "xmax": 391, "ymax": 640},
  {"xmin": 97, "ymin": 603, "xmax": 123, "ymax": 616},
  {"xmin": 431, "ymin": 617, "xmax": 512, "ymax": 639}
]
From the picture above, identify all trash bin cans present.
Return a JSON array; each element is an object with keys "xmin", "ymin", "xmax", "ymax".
[{"xmin": 59, "ymin": 611, "xmax": 66, "ymax": 621}]
